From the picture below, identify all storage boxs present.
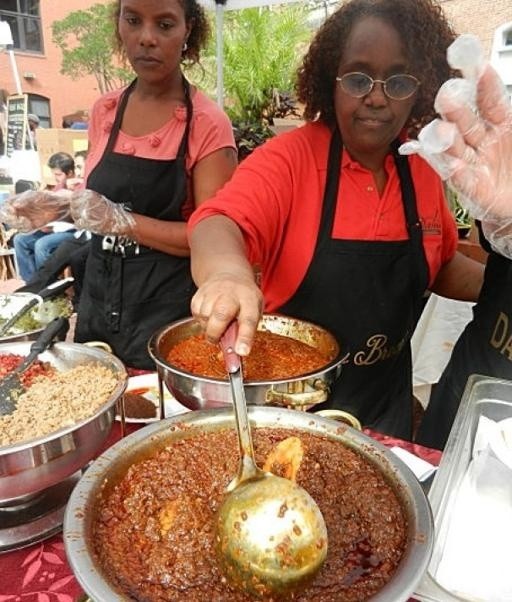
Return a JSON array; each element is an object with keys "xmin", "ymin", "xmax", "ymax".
[
  {"xmin": 402, "ymin": 375, "xmax": 512, "ymax": 600},
  {"xmin": 36, "ymin": 128, "xmax": 88, "ymax": 184}
]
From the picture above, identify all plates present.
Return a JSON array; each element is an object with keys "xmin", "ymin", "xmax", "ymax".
[{"xmin": 112, "ymin": 371, "xmax": 192, "ymax": 423}]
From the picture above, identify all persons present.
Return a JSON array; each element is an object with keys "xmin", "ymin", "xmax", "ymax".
[
  {"xmin": 4, "ymin": 0, "xmax": 239, "ymax": 369},
  {"xmin": 0, "ymin": 8, "xmax": 89, "ymax": 282},
  {"xmin": 188, "ymin": 0, "xmax": 488, "ymax": 439},
  {"xmin": 414, "ymin": 60, "xmax": 512, "ymax": 450}
]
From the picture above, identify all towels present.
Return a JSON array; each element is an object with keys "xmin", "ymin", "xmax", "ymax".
[{"xmin": 410, "ymin": 291, "xmax": 478, "ymax": 412}]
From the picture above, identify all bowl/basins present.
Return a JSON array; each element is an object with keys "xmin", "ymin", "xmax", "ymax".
[{"xmin": 0, "ymin": 292, "xmax": 74, "ymax": 343}]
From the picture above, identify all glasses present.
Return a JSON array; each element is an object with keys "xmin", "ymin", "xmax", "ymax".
[{"xmin": 333, "ymin": 70, "xmax": 424, "ymax": 100}]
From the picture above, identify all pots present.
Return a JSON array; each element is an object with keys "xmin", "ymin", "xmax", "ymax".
[
  {"xmin": 60, "ymin": 408, "xmax": 437, "ymax": 602},
  {"xmin": 1, "ymin": 338, "xmax": 130, "ymax": 556},
  {"xmin": 146, "ymin": 313, "xmax": 351, "ymax": 411}
]
